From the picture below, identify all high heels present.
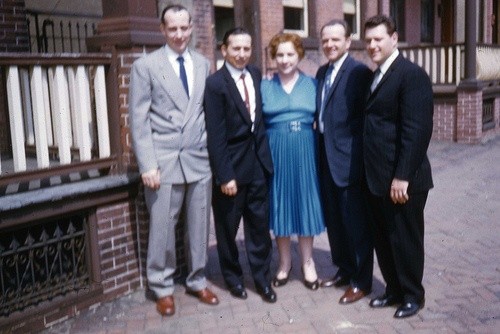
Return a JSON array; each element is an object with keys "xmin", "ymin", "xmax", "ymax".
[
  {"xmin": 273, "ymin": 263, "xmax": 294, "ymax": 287},
  {"xmin": 299, "ymin": 265, "xmax": 320, "ymax": 290}
]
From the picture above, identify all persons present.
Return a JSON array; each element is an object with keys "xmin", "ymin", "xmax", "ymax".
[
  {"xmin": 128, "ymin": 5, "xmax": 218, "ymax": 316},
  {"xmin": 356, "ymin": 15, "xmax": 435, "ymax": 317},
  {"xmin": 315, "ymin": 19, "xmax": 375, "ymax": 303},
  {"xmin": 203, "ymin": 27, "xmax": 277, "ymax": 301},
  {"xmin": 260, "ymin": 33, "xmax": 326, "ymax": 289}
]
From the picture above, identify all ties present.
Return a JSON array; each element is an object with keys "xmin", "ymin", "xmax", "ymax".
[
  {"xmin": 239, "ymin": 74, "xmax": 251, "ymax": 115},
  {"xmin": 177, "ymin": 56, "xmax": 190, "ymax": 101},
  {"xmin": 370, "ymin": 69, "xmax": 383, "ymax": 92},
  {"xmin": 324, "ymin": 64, "xmax": 334, "ymax": 95}
]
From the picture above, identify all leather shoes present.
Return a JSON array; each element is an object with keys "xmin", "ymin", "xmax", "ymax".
[
  {"xmin": 338, "ymin": 286, "xmax": 372, "ymax": 303},
  {"xmin": 155, "ymin": 295, "xmax": 176, "ymax": 316},
  {"xmin": 184, "ymin": 289, "xmax": 220, "ymax": 305},
  {"xmin": 259, "ymin": 285, "xmax": 277, "ymax": 302},
  {"xmin": 370, "ymin": 293, "xmax": 406, "ymax": 308},
  {"xmin": 228, "ymin": 284, "xmax": 249, "ymax": 299},
  {"xmin": 395, "ymin": 301, "xmax": 425, "ymax": 318},
  {"xmin": 319, "ymin": 274, "xmax": 349, "ymax": 288}
]
